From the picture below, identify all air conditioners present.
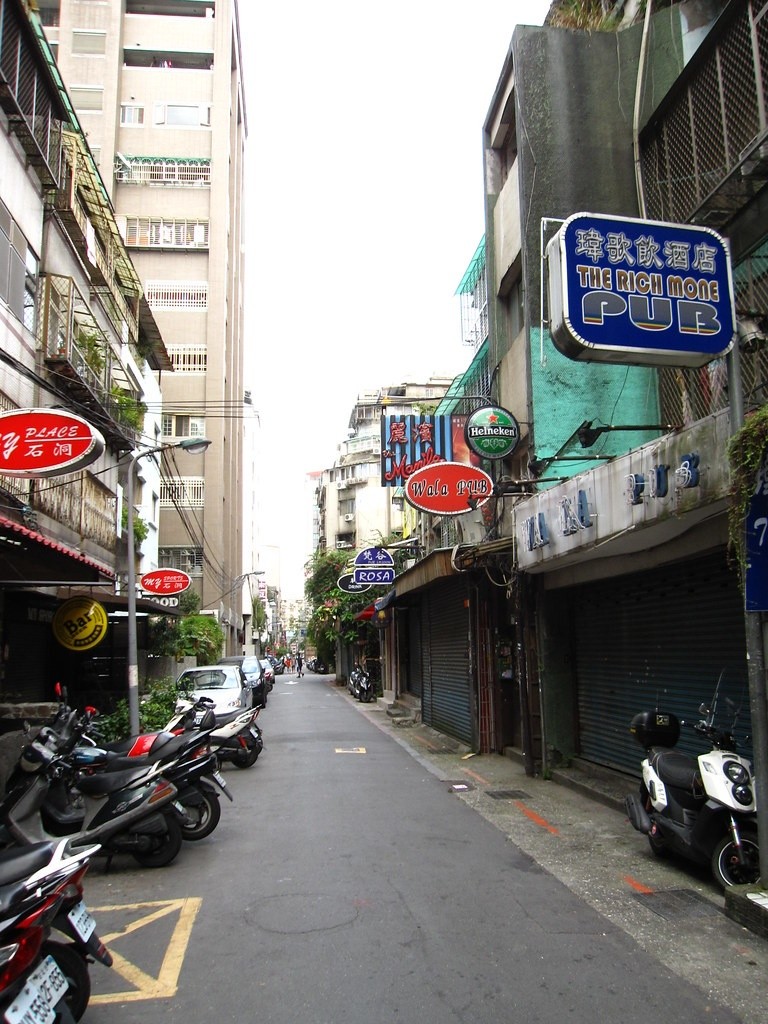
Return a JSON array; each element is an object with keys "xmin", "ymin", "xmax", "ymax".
[
  {"xmin": 335, "ymin": 475, "xmax": 368, "ymax": 489},
  {"xmin": 344, "ymin": 513, "xmax": 354, "ymax": 523},
  {"xmin": 336, "ymin": 540, "xmax": 348, "ymax": 549},
  {"xmin": 373, "ymin": 448, "xmax": 381, "ymax": 454}
]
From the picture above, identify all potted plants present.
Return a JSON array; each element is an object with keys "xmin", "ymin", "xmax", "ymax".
[
  {"xmin": 400, "ymin": 551, "xmax": 416, "ymax": 572},
  {"xmin": 46, "ymin": 324, "xmax": 145, "ymax": 445}
]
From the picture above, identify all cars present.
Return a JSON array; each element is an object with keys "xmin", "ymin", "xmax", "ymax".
[
  {"xmin": 217, "ymin": 655, "xmax": 268, "ymax": 709},
  {"xmin": 258, "ymin": 658, "xmax": 276, "ymax": 685},
  {"xmin": 267, "ymin": 653, "xmax": 286, "ymax": 674},
  {"xmin": 173, "ymin": 663, "xmax": 254, "ymax": 728}
]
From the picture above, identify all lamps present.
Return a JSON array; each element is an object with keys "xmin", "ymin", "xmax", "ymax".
[
  {"xmin": 736, "ymin": 317, "xmax": 766, "ymax": 353},
  {"xmin": 467, "ymin": 419, "xmax": 683, "ymax": 512}
]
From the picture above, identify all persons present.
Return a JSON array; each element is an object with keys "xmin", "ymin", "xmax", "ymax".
[{"xmin": 284, "ymin": 653, "xmax": 305, "ymax": 678}]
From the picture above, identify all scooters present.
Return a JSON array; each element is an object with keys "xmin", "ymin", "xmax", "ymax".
[
  {"xmin": 620, "ymin": 668, "xmax": 763, "ymax": 894},
  {"xmin": 349, "ymin": 662, "xmax": 374, "ymax": 703},
  {"xmin": 0, "ymin": 680, "xmax": 268, "ymax": 1024}
]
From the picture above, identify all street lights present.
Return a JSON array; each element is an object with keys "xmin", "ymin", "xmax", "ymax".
[
  {"xmin": 126, "ymin": 436, "xmax": 214, "ymax": 737},
  {"xmin": 233, "ymin": 570, "xmax": 266, "ymax": 656},
  {"xmin": 266, "ymin": 620, "xmax": 283, "ymax": 655}
]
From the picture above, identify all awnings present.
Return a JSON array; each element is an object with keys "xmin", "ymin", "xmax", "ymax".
[
  {"xmin": 355, "ymin": 537, "xmax": 512, "ymax": 620},
  {"xmin": 0, "ymin": 512, "xmax": 179, "ymax": 617}
]
